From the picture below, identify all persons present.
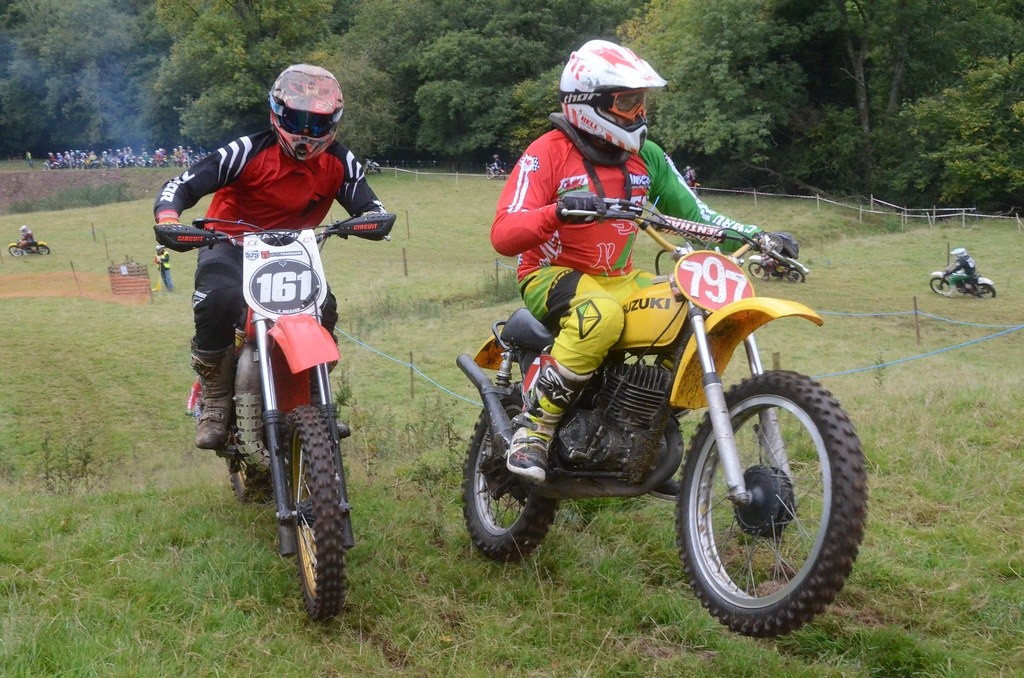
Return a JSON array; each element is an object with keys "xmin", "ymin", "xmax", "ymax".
[
  {"xmin": 493, "ymin": 154, "xmax": 501, "ymax": 174},
  {"xmin": 761, "ymin": 256, "xmax": 776, "ymax": 281},
  {"xmin": 944, "ymin": 248, "xmax": 975, "ymax": 293},
  {"xmin": 47, "ymin": 145, "xmax": 207, "ymax": 168},
  {"xmin": 366, "ymin": 159, "xmax": 373, "ymax": 173},
  {"xmin": 155, "ymin": 64, "xmax": 392, "ymax": 448},
  {"xmin": 26, "ymin": 151, "xmax": 32, "ymax": 168},
  {"xmin": 682, "ymin": 166, "xmax": 697, "ymax": 187},
  {"xmin": 490, "ymin": 40, "xmax": 798, "ymax": 498},
  {"xmin": 154, "ymin": 245, "xmax": 173, "ymax": 290},
  {"xmin": 16, "ymin": 226, "xmax": 32, "ymax": 255}
]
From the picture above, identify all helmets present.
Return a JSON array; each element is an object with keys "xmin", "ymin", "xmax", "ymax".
[
  {"xmin": 20, "ymin": 226, "xmax": 28, "ymax": 232},
  {"xmin": 950, "ymin": 248, "xmax": 968, "ymax": 260},
  {"xmin": 269, "ymin": 64, "xmax": 345, "ymax": 161},
  {"xmin": 560, "ymin": 38, "xmax": 668, "ymax": 154}
]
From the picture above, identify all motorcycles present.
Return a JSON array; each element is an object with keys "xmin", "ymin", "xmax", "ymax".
[
  {"xmin": 686, "ymin": 181, "xmax": 703, "ymax": 195},
  {"xmin": 8, "ymin": 239, "xmax": 51, "ymax": 257},
  {"xmin": 156, "ymin": 212, "xmax": 397, "ymax": 622},
  {"xmin": 452, "ymin": 196, "xmax": 870, "ymax": 640},
  {"xmin": 747, "ymin": 248, "xmax": 806, "ymax": 285},
  {"xmin": 929, "ymin": 269, "xmax": 997, "ymax": 300},
  {"xmin": 485, "ymin": 162, "xmax": 506, "ymax": 181}
]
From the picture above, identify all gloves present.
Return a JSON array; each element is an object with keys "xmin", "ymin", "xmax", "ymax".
[
  {"xmin": 757, "ymin": 230, "xmax": 802, "ymax": 269},
  {"xmin": 557, "ymin": 188, "xmax": 607, "ymax": 227}
]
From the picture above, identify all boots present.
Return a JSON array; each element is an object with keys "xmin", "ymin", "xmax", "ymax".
[
  {"xmin": 650, "ymin": 478, "xmax": 678, "ymax": 500},
  {"xmin": 337, "ymin": 418, "xmax": 351, "ymax": 440},
  {"xmin": 192, "ymin": 338, "xmax": 236, "ymax": 450},
  {"xmin": 507, "ymin": 345, "xmax": 595, "ymax": 483}
]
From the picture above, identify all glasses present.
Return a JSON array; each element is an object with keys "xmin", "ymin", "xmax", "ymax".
[
  {"xmin": 608, "ymin": 84, "xmax": 649, "ymax": 124},
  {"xmin": 280, "ymin": 102, "xmax": 333, "ymax": 137}
]
